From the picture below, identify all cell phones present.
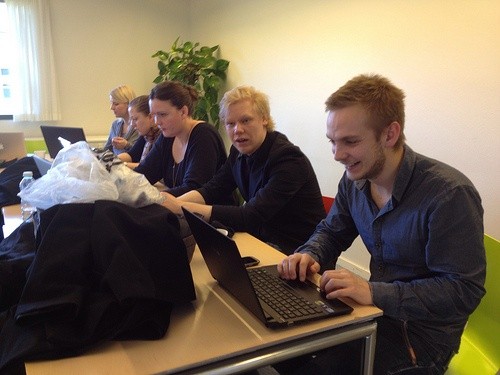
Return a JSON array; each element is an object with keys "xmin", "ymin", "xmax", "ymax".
[{"xmin": 242, "ymin": 256, "xmax": 260, "ymax": 266}]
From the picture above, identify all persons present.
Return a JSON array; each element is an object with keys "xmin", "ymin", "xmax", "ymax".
[
  {"xmin": 133, "ymin": 83, "xmax": 238, "ymax": 206},
  {"xmin": 277, "ymin": 75, "xmax": 487, "ymax": 375},
  {"xmin": 104, "ymin": 86, "xmax": 139, "ymax": 156},
  {"xmin": 118, "ymin": 95, "xmax": 161, "ymax": 167},
  {"xmin": 159, "ymin": 86, "xmax": 326, "ymax": 256}
]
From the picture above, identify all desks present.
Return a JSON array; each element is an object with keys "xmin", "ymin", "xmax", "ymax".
[{"xmin": 2, "ymin": 203, "xmax": 384, "ymax": 375}]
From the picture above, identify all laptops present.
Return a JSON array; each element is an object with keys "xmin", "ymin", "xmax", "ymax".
[
  {"xmin": 0, "ymin": 133, "xmax": 27, "ymax": 162},
  {"xmin": 40, "ymin": 125, "xmax": 86, "ymax": 159},
  {"xmin": 181, "ymin": 206, "xmax": 354, "ymax": 329}
]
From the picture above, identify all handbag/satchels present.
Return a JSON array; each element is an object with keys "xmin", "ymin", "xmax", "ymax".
[{"xmin": 17, "ymin": 141, "xmax": 167, "ymax": 212}]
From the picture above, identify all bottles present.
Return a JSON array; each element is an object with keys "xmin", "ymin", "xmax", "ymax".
[{"xmin": 19, "ymin": 171, "xmax": 35, "ymax": 222}]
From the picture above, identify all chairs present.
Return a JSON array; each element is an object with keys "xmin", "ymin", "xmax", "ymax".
[
  {"xmin": 0, "ymin": 132, "xmax": 27, "ymax": 164},
  {"xmin": 445, "ymin": 233, "xmax": 500, "ymax": 375}
]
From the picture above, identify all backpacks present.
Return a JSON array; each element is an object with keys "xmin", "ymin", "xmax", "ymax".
[{"xmin": 0, "ymin": 156, "xmax": 41, "ymax": 207}]
